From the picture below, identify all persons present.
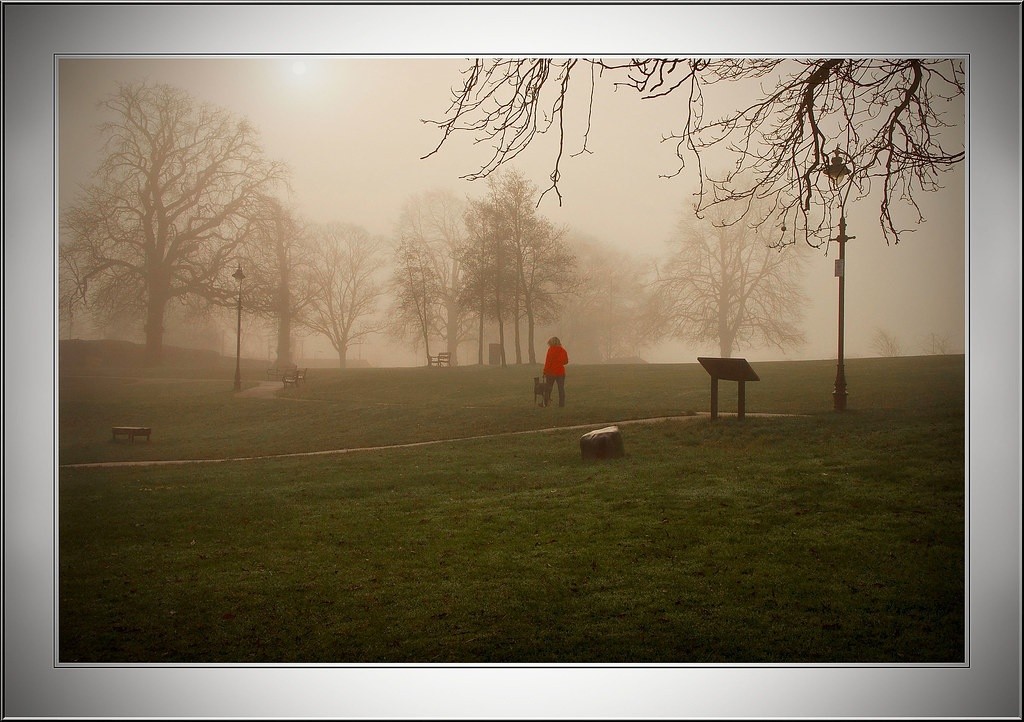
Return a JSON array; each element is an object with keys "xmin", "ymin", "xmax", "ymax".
[{"xmin": 538, "ymin": 336, "xmax": 568, "ymax": 408}]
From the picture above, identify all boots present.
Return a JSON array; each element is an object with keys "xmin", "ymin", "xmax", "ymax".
[
  {"xmin": 538, "ymin": 389, "xmax": 552, "ymax": 407},
  {"xmin": 559, "ymin": 391, "xmax": 565, "ymax": 407}
]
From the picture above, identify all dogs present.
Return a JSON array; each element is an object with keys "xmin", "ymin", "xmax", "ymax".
[{"xmin": 533, "ymin": 377, "xmax": 553, "ymax": 407}]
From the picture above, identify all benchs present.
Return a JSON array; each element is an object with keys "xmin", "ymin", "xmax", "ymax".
[
  {"xmin": 281, "ymin": 368, "xmax": 307, "ymax": 387},
  {"xmin": 267, "ymin": 365, "xmax": 297, "ymax": 381},
  {"xmin": 112, "ymin": 427, "xmax": 151, "ymax": 442},
  {"xmin": 428, "ymin": 352, "xmax": 452, "ymax": 368}
]
